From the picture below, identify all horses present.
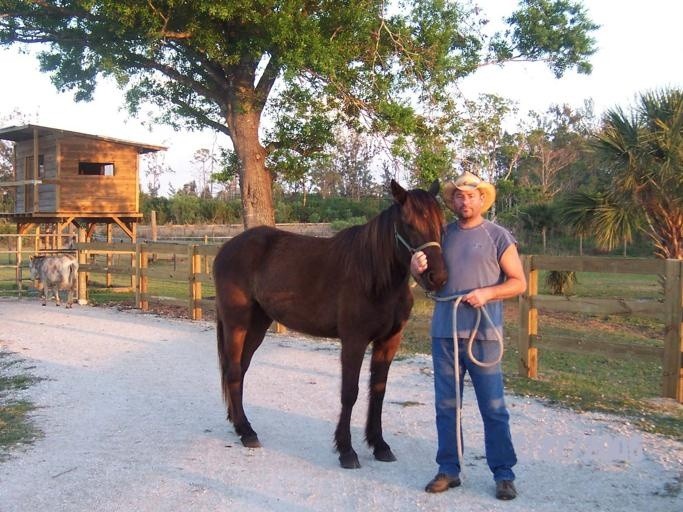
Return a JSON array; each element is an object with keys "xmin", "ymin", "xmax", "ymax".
[
  {"xmin": 28, "ymin": 254, "xmax": 78, "ymax": 309},
  {"xmin": 211, "ymin": 177, "xmax": 449, "ymax": 470}
]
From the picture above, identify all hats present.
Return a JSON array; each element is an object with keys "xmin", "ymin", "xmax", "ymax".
[{"xmin": 441, "ymin": 171, "xmax": 496, "ymax": 214}]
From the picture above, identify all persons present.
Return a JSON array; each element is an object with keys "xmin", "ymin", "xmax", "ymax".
[{"xmin": 408, "ymin": 171, "xmax": 527, "ymax": 501}]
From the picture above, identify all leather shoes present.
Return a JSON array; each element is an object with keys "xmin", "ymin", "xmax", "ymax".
[
  {"xmin": 425, "ymin": 473, "xmax": 460, "ymax": 493},
  {"xmin": 496, "ymin": 480, "xmax": 516, "ymax": 500}
]
